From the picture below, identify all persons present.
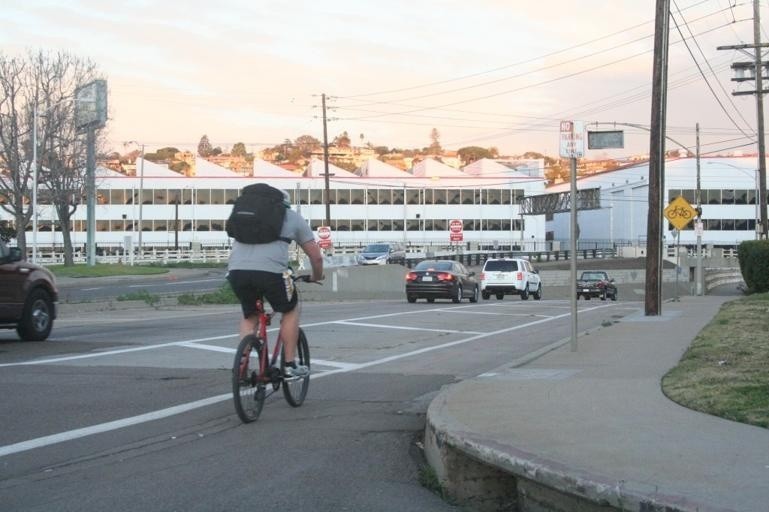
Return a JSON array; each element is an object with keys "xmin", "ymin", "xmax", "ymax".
[{"xmin": 224, "ymin": 190, "xmax": 326, "ymax": 386}]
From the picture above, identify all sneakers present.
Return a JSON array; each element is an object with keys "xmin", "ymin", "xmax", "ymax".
[
  {"xmin": 284, "ymin": 364, "xmax": 309, "ymax": 383},
  {"xmin": 233, "ymin": 372, "xmax": 251, "ymax": 383}
]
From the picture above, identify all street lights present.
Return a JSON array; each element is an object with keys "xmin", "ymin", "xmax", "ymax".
[
  {"xmin": 129, "ymin": 140, "xmax": 144, "ymax": 255},
  {"xmin": 32, "ymin": 98, "xmax": 95, "ymax": 261},
  {"xmin": 707, "ymin": 161, "xmax": 758, "ymax": 240}
]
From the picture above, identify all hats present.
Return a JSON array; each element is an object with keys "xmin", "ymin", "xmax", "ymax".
[{"xmin": 279, "ymin": 189, "xmax": 291, "ymax": 208}]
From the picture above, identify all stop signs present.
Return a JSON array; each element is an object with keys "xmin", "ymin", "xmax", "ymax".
[
  {"xmin": 317, "ymin": 227, "xmax": 332, "ymax": 240},
  {"xmin": 450, "ymin": 220, "xmax": 463, "ymax": 233}
]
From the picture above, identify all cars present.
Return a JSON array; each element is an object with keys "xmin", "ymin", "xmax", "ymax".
[
  {"xmin": 405, "ymin": 260, "xmax": 479, "ymax": 303},
  {"xmin": 0, "ymin": 235, "xmax": 58, "ymax": 341},
  {"xmin": 480, "ymin": 258, "xmax": 542, "ymax": 300}
]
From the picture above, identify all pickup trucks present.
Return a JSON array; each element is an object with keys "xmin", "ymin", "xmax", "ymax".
[{"xmin": 576, "ymin": 271, "xmax": 617, "ymax": 300}]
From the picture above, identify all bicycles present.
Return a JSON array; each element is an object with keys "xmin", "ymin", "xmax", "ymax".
[{"xmin": 232, "ymin": 267, "xmax": 323, "ymax": 422}]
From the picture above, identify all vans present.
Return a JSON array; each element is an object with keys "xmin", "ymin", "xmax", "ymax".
[{"xmin": 357, "ymin": 242, "xmax": 406, "ymax": 265}]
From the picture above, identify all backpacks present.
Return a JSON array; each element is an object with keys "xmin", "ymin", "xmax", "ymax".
[{"xmin": 226, "ymin": 184, "xmax": 286, "ymax": 244}]
